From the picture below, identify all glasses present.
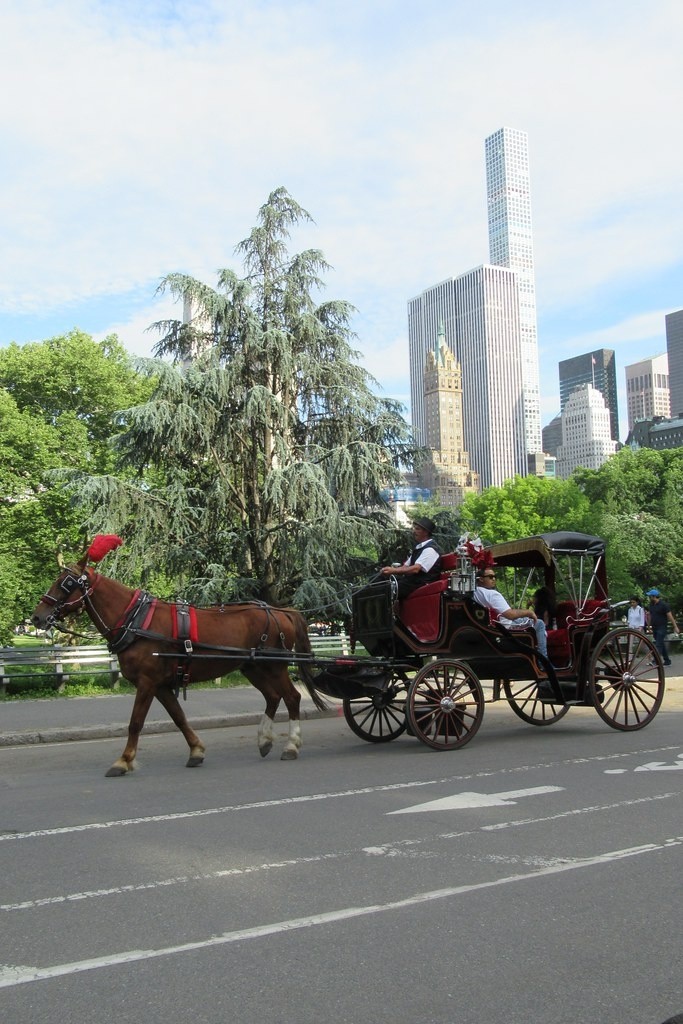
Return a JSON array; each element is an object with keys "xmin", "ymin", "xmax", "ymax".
[{"xmin": 483, "ymin": 574, "xmax": 497, "ymax": 579}]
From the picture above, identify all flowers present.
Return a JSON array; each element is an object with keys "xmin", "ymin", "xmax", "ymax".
[{"xmin": 462, "ymin": 538, "xmax": 498, "ymax": 571}]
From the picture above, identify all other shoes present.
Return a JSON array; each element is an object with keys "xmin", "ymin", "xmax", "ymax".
[
  {"xmin": 649, "ymin": 661, "xmax": 656, "ymax": 665},
  {"xmin": 540, "ymin": 662, "xmax": 555, "ymax": 670},
  {"xmin": 663, "ymin": 661, "xmax": 672, "ymax": 667}
]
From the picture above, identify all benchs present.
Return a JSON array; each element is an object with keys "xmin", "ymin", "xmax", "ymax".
[{"xmin": 0, "ymin": 552, "xmax": 683, "ymax": 694}]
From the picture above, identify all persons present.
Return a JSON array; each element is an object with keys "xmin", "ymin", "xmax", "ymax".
[
  {"xmin": 627, "ymin": 590, "xmax": 679, "ymax": 667},
  {"xmin": 380, "ymin": 517, "xmax": 443, "ymax": 612},
  {"xmin": 526, "ymin": 587, "xmax": 555, "ymax": 630},
  {"xmin": 472, "ymin": 568, "xmax": 556, "ymax": 670}
]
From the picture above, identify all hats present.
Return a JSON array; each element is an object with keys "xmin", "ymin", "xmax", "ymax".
[
  {"xmin": 413, "ymin": 517, "xmax": 437, "ymax": 537},
  {"xmin": 646, "ymin": 590, "xmax": 660, "ymax": 597}
]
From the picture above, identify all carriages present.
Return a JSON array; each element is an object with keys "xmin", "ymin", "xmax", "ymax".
[{"xmin": 29, "ymin": 529, "xmax": 665, "ymax": 777}]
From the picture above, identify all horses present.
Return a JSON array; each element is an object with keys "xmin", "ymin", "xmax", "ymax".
[{"xmin": 30, "ymin": 544, "xmax": 336, "ymax": 778}]
